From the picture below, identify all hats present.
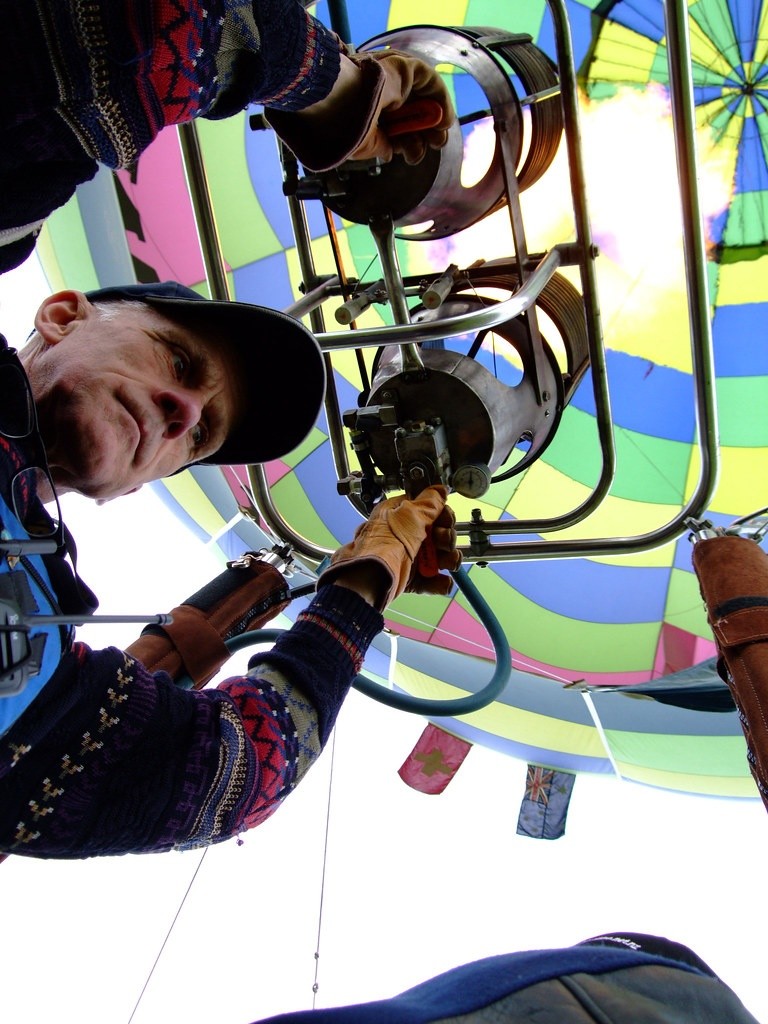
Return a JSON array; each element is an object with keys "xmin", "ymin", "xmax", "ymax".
[{"xmin": 25, "ymin": 281, "xmax": 327, "ymax": 478}]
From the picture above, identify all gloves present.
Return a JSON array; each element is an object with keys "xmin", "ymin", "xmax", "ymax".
[
  {"xmin": 315, "ymin": 486, "xmax": 464, "ymax": 615},
  {"xmin": 263, "ymin": 48, "xmax": 455, "ymax": 172}
]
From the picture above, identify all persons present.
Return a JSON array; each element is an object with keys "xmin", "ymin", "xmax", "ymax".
[{"xmin": 0, "ymin": 0, "xmax": 464, "ymax": 858}]
